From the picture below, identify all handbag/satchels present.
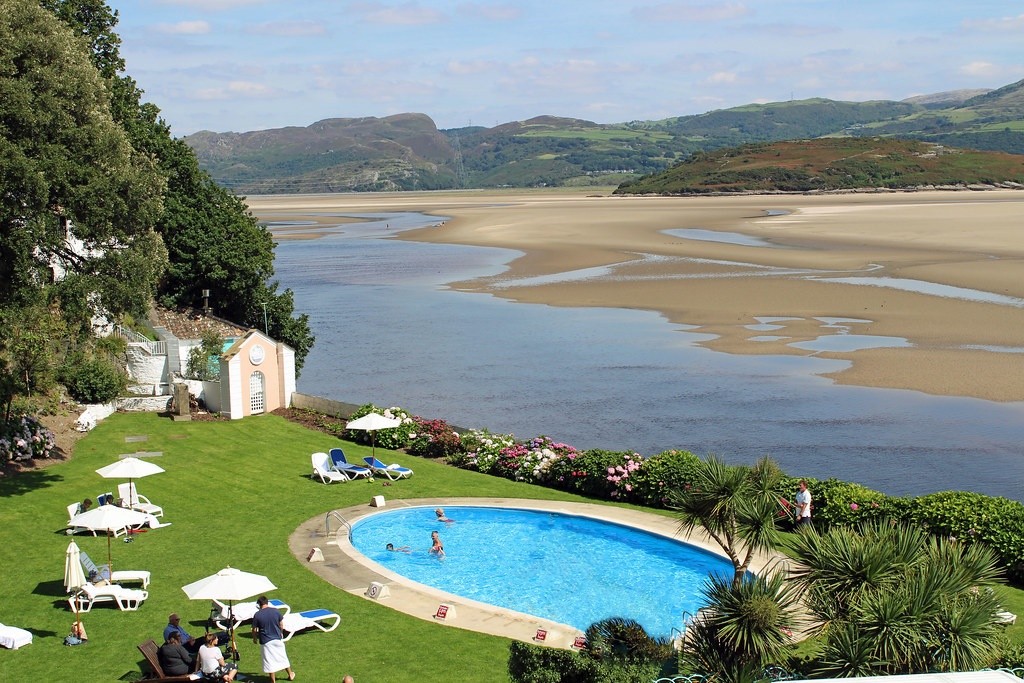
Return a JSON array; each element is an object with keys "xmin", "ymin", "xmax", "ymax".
[{"xmin": 71, "ymin": 621, "xmax": 87, "ymax": 639}]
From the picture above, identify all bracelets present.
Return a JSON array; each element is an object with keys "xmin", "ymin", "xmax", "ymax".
[{"xmin": 799, "ymin": 514, "xmax": 802, "ymax": 517}]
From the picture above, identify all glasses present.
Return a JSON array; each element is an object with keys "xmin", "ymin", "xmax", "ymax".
[{"xmin": 171, "ymin": 618, "xmax": 181, "ymax": 621}]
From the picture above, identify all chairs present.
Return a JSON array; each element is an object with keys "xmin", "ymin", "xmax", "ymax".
[
  {"xmin": 67, "ymin": 492, "xmax": 150, "ymax": 538},
  {"xmin": 211, "ymin": 599, "xmax": 291, "ymax": 631},
  {"xmin": 137, "ymin": 640, "xmax": 205, "ymax": 683},
  {"xmin": 329, "ymin": 448, "xmax": 372, "ymax": 481},
  {"xmin": 68, "ymin": 582, "xmax": 148, "ymax": 613},
  {"xmin": 311, "ymin": 452, "xmax": 347, "ymax": 485},
  {"xmin": 0, "ymin": 622, "xmax": 33, "ymax": 650},
  {"xmin": 283, "ymin": 609, "xmax": 341, "ymax": 641},
  {"xmin": 80, "ymin": 552, "xmax": 151, "ymax": 589},
  {"xmin": 363, "ymin": 457, "xmax": 414, "ymax": 481},
  {"xmin": 118, "ymin": 482, "xmax": 163, "ymax": 518}
]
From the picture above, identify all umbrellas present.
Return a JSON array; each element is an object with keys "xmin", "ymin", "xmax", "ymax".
[
  {"xmin": 95, "ymin": 458, "xmax": 165, "ymax": 507},
  {"xmin": 64, "ymin": 538, "xmax": 87, "ymax": 638},
  {"xmin": 345, "ymin": 413, "xmax": 400, "ymax": 470},
  {"xmin": 67, "ymin": 504, "xmax": 152, "ymax": 585},
  {"xmin": 181, "ymin": 565, "xmax": 278, "ymax": 663}
]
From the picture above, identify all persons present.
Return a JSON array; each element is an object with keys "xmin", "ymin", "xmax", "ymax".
[
  {"xmin": 343, "ymin": 676, "xmax": 354, "ymax": 683},
  {"xmin": 159, "ymin": 631, "xmax": 197, "ymax": 676},
  {"xmin": 163, "ymin": 613, "xmax": 206, "ymax": 654},
  {"xmin": 75, "ymin": 499, "xmax": 91, "ymax": 515},
  {"xmin": 432, "ymin": 530, "xmax": 442, "ymax": 547},
  {"xmin": 252, "ymin": 596, "xmax": 295, "ymax": 683},
  {"xmin": 195, "ymin": 633, "xmax": 237, "ymax": 683},
  {"xmin": 794, "ymin": 480, "xmax": 812, "ymax": 529},
  {"xmin": 106, "ymin": 495, "xmax": 113, "ymax": 505},
  {"xmin": 435, "ymin": 507, "xmax": 444, "ymax": 520}
]
